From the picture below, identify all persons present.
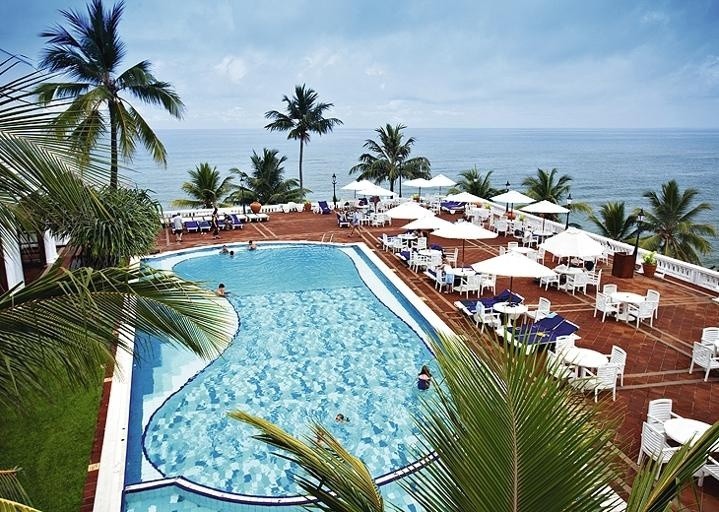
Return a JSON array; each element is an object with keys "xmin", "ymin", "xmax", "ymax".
[
  {"xmin": 216, "ymin": 283, "xmax": 225, "ymax": 296},
  {"xmin": 249, "ymin": 240, "xmax": 256, "ymax": 250},
  {"xmin": 220, "ymin": 245, "xmax": 228, "ymax": 253},
  {"xmin": 230, "ymin": 251, "xmax": 234, "ymax": 255},
  {"xmin": 210, "ymin": 208, "xmax": 233, "ymax": 238},
  {"xmin": 418, "ymin": 364, "xmax": 431, "ymax": 390},
  {"xmin": 438, "ymin": 254, "xmax": 454, "ymax": 294},
  {"xmin": 174, "ymin": 213, "xmax": 184, "ymax": 242},
  {"xmin": 415, "ymin": 229, "xmax": 423, "ymax": 242},
  {"xmin": 336, "ymin": 413, "xmax": 344, "ymax": 423},
  {"xmin": 334, "ymin": 208, "xmax": 362, "ymax": 238},
  {"xmin": 316, "ymin": 431, "xmax": 325, "ymax": 444}
]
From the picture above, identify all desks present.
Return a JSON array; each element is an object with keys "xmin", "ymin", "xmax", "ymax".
[{"xmin": 664, "ymin": 419, "xmax": 718, "ymax": 452}]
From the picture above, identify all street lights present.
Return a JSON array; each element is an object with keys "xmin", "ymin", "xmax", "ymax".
[
  {"xmin": 632, "ymin": 207, "xmax": 646, "ymax": 262},
  {"xmin": 240, "ymin": 176, "xmax": 246, "ymax": 214},
  {"xmin": 564, "ymin": 191, "xmax": 573, "ymax": 231},
  {"xmin": 472, "ymin": 175, "xmax": 479, "ymax": 195},
  {"xmin": 331, "ymin": 173, "xmax": 337, "ymax": 203},
  {"xmin": 504, "ymin": 180, "xmax": 510, "ymax": 213}
]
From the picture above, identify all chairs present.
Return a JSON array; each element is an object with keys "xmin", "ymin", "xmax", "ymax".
[
  {"xmin": 698, "ymin": 454, "xmax": 718, "ymax": 487},
  {"xmin": 500, "ymin": 232, "xmax": 660, "ymax": 329},
  {"xmin": 330, "ymin": 190, "xmax": 562, "ymax": 300},
  {"xmin": 166, "ymin": 201, "xmax": 337, "ymax": 236},
  {"xmin": 647, "ymin": 399, "xmax": 684, "ymax": 439},
  {"xmin": 702, "ymin": 327, "xmax": 719, "ymax": 357},
  {"xmin": 454, "ymin": 290, "xmax": 627, "ymax": 403},
  {"xmin": 688, "ymin": 341, "xmax": 718, "ymax": 382},
  {"xmin": 637, "ymin": 422, "xmax": 680, "ymax": 480}
]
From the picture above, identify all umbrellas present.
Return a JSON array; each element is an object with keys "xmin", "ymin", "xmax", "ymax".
[
  {"xmin": 386, "ymin": 202, "xmax": 436, "ymax": 222},
  {"xmin": 341, "ymin": 179, "xmax": 396, "ymax": 198},
  {"xmin": 429, "ymin": 219, "xmax": 499, "ymax": 267},
  {"xmin": 430, "ymin": 173, "xmax": 457, "ymax": 195},
  {"xmin": 403, "ymin": 178, "xmax": 433, "ymax": 197},
  {"xmin": 443, "ymin": 191, "xmax": 488, "ymax": 219},
  {"xmin": 490, "ymin": 190, "xmax": 537, "ymax": 222},
  {"xmin": 472, "ymin": 250, "xmax": 555, "ymax": 306},
  {"xmin": 400, "ymin": 216, "xmax": 453, "ymax": 230},
  {"xmin": 519, "ymin": 200, "xmax": 570, "ymax": 231},
  {"xmin": 540, "ymin": 226, "xmax": 607, "ymax": 269}
]
